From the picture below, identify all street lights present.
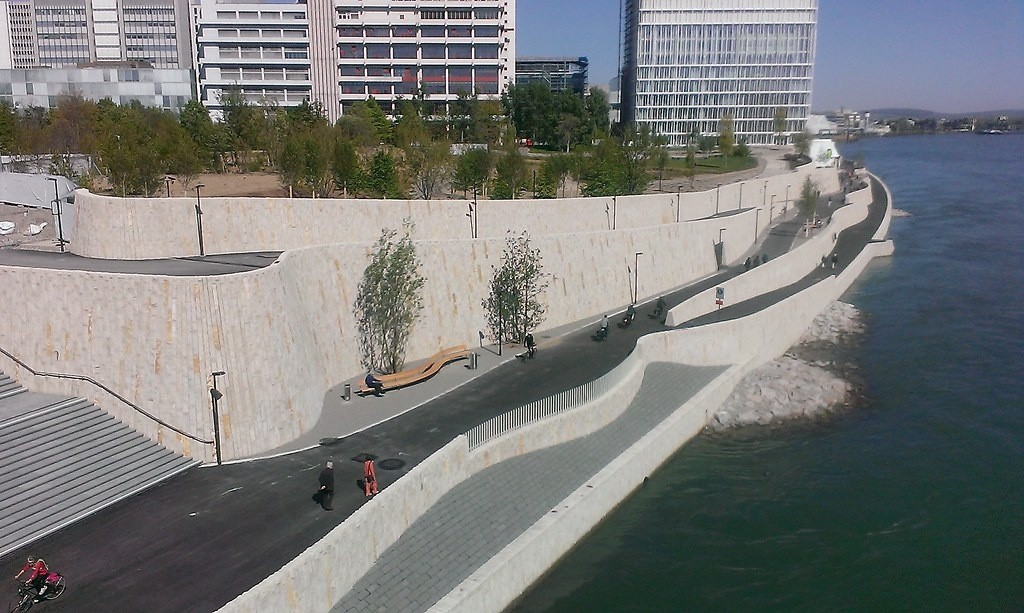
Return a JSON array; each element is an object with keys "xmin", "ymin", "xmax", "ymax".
[
  {"xmin": 718, "ymin": 228, "xmax": 726, "ymax": 271},
  {"xmin": 786, "ymin": 185, "xmax": 791, "ymax": 209},
  {"xmin": 44, "ymin": 178, "xmax": 65, "ymax": 253},
  {"xmin": 677, "ymin": 186, "xmax": 684, "ymax": 221},
  {"xmin": 764, "ymin": 181, "xmax": 769, "ymax": 205},
  {"xmin": 613, "ymin": 192, "xmax": 619, "ymax": 230},
  {"xmin": 195, "ymin": 184, "xmax": 205, "ymax": 256},
  {"xmin": 739, "ymin": 182, "xmax": 746, "ymax": 210},
  {"xmin": 770, "ymin": 195, "xmax": 776, "ymax": 223},
  {"xmin": 212, "ymin": 371, "xmax": 226, "ymax": 465},
  {"xmin": 755, "ymin": 208, "xmax": 762, "ymax": 245},
  {"xmin": 634, "ymin": 253, "xmax": 644, "ymax": 305},
  {"xmin": 716, "ymin": 184, "xmax": 722, "ymax": 214},
  {"xmin": 472, "ymin": 188, "xmax": 481, "ymax": 239}
]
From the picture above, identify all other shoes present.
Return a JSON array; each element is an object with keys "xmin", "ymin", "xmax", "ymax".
[
  {"xmin": 34, "ymin": 597, "xmax": 39, "ymax": 603},
  {"xmin": 39, "ymin": 585, "xmax": 47, "ymax": 595}
]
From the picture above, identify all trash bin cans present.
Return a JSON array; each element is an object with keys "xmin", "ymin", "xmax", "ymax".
[
  {"xmin": 344, "ymin": 384, "xmax": 350, "ymax": 401},
  {"xmin": 469, "ymin": 352, "xmax": 477, "ymax": 370}
]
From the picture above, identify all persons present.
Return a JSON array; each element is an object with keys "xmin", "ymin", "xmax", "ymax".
[
  {"xmin": 832, "ymin": 254, "xmax": 838, "ymax": 269},
  {"xmin": 364, "ymin": 456, "xmax": 379, "ymax": 496},
  {"xmin": 628, "ymin": 303, "xmax": 636, "ymax": 320},
  {"xmin": 365, "ymin": 370, "xmax": 385, "ymax": 397},
  {"xmin": 600, "ymin": 314, "xmax": 610, "ymax": 336},
  {"xmin": 523, "ymin": 332, "xmax": 534, "ymax": 359},
  {"xmin": 14, "ymin": 555, "xmax": 49, "ymax": 603},
  {"xmin": 319, "ymin": 461, "xmax": 334, "ymax": 511},
  {"xmin": 657, "ymin": 295, "xmax": 666, "ymax": 316},
  {"xmin": 744, "ymin": 255, "xmax": 768, "ymax": 271},
  {"xmin": 822, "ymin": 255, "xmax": 826, "ymax": 268}
]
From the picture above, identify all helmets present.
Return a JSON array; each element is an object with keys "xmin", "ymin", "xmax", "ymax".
[{"xmin": 28, "ymin": 555, "xmax": 38, "ymax": 562}]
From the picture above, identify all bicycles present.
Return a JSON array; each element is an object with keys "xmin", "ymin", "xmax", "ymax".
[
  {"xmin": 601, "ymin": 329, "xmax": 607, "ymax": 338},
  {"xmin": 625, "ymin": 314, "xmax": 635, "ymax": 326},
  {"xmin": 525, "ymin": 344, "xmax": 537, "ymax": 360},
  {"xmin": 11, "ymin": 572, "xmax": 65, "ymax": 613},
  {"xmin": 654, "ymin": 304, "xmax": 668, "ymax": 320}
]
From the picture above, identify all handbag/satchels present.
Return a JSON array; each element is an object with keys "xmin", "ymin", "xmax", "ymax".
[{"xmin": 367, "ymin": 475, "xmax": 373, "ymax": 482}]
